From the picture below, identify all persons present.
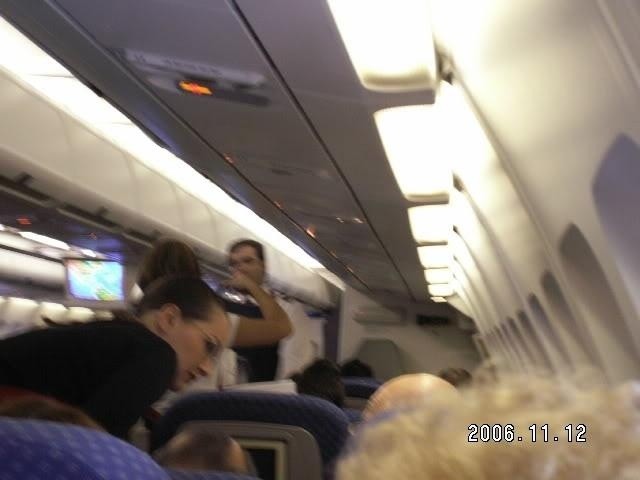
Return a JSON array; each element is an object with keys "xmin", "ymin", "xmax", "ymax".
[
  {"xmin": 335, "ymin": 365, "xmax": 639, "ymax": 479},
  {"xmin": 0, "ymin": 239, "xmax": 373, "ymax": 479}
]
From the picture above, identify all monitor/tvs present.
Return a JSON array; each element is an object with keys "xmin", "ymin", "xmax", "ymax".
[
  {"xmin": 237, "ymin": 438, "xmax": 288, "ymax": 480},
  {"xmin": 64, "ymin": 256, "xmax": 127, "ymax": 309}
]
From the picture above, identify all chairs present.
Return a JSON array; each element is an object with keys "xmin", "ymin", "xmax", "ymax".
[{"xmin": 0, "ymin": 366, "xmax": 422, "ymax": 480}]
